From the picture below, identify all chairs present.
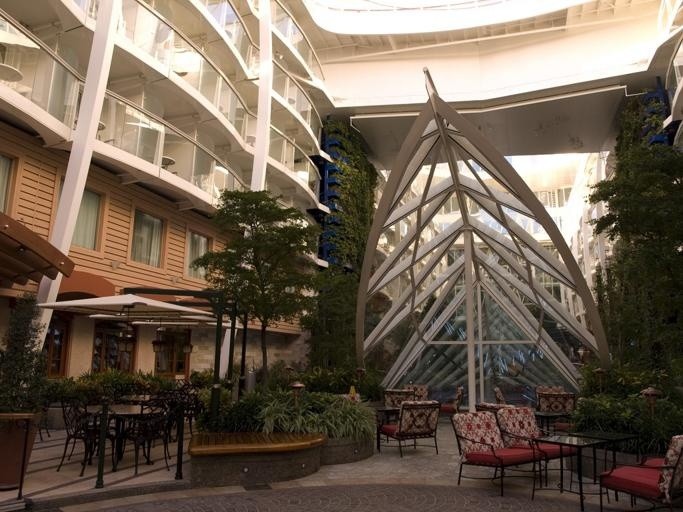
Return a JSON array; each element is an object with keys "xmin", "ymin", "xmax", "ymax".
[
  {"xmin": 383, "ymin": 388, "xmax": 415, "ymax": 444},
  {"xmin": 337, "ymin": 392, "xmax": 362, "ymax": 405},
  {"xmin": 474, "ymin": 384, "xmax": 577, "ymax": 411},
  {"xmin": 450, "ymin": 410, "xmax": 540, "ymax": 497},
  {"xmin": 453, "ymin": 386, "xmax": 471, "ymax": 412},
  {"xmin": 404, "ymin": 384, "xmax": 430, "ymax": 401},
  {"xmin": 37, "ymin": 380, "xmax": 206, "ymax": 478},
  {"xmin": 600, "ymin": 434, "xmax": 680, "ymax": 511},
  {"xmin": 496, "ymin": 405, "xmax": 581, "ymax": 488}
]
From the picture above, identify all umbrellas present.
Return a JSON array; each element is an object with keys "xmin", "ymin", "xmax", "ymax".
[
  {"xmin": 86, "ymin": 304, "xmax": 232, "ymax": 368},
  {"xmin": 33, "ymin": 292, "xmax": 215, "ymax": 386}
]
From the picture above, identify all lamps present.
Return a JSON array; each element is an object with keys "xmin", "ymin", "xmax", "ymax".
[{"xmin": 120, "ymin": 305, "xmax": 194, "ymax": 354}]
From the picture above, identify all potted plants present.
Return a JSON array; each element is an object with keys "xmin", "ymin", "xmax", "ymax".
[{"xmin": 1, "ymin": 291, "xmax": 50, "ymax": 491}]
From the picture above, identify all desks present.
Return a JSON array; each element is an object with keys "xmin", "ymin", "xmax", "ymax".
[
  {"xmin": 161, "ymin": 157, "xmax": 176, "ymax": 170},
  {"xmin": 570, "ymin": 430, "xmax": 643, "ymax": 509},
  {"xmin": 0, "ymin": 64, "xmax": 22, "ymax": 85},
  {"xmin": 533, "ymin": 411, "xmax": 570, "ymax": 435},
  {"xmin": 75, "ymin": 120, "xmax": 105, "ymax": 139},
  {"xmin": 369, "ymin": 407, "xmax": 400, "ymax": 454},
  {"xmin": 529, "ymin": 435, "xmax": 608, "ymax": 511}
]
